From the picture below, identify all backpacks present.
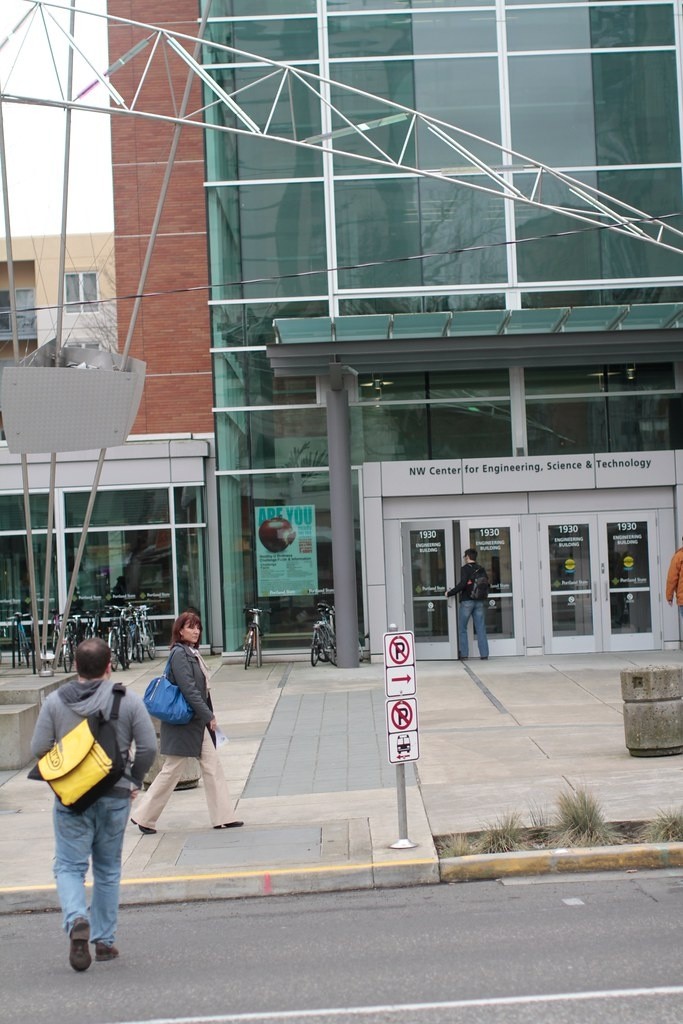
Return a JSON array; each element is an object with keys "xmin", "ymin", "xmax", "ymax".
[{"xmin": 466, "ymin": 564, "xmax": 489, "ymax": 600}]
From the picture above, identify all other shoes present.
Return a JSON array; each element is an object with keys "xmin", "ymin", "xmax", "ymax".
[
  {"xmin": 460, "ymin": 657, "xmax": 468, "ymax": 660},
  {"xmin": 95, "ymin": 943, "xmax": 119, "ymax": 961},
  {"xmin": 480, "ymin": 657, "xmax": 488, "ymax": 660},
  {"xmin": 69, "ymin": 917, "xmax": 92, "ymax": 972}
]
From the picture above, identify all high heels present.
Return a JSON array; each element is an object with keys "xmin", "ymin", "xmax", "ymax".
[
  {"xmin": 130, "ymin": 818, "xmax": 157, "ymax": 834},
  {"xmin": 214, "ymin": 821, "xmax": 244, "ymax": 829}
]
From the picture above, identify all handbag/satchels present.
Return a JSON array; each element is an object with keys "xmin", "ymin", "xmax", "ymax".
[
  {"xmin": 143, "ymin": 677, "xmax": 194, "ymax": 724},
  {"xmin": 27, "ymin": 709, "xmax": 125, "ymax": 817}
]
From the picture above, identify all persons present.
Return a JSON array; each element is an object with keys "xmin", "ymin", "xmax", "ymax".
[
  {"xmin": 666, "ymin": 536, "xmax": 683, "ymax": 616},
  {"xmin": 111, "ymin": 576, "xmax": 126, "ymax": 606},
  {"xmin": 130, "ymin": 612, "xmax": 244, "ymax": 835},
  {"xmin": 32, "ymin": 638, "xmax": 156, "ymax": 970},
  {"xmin": 446, "ymin": 548, "xmax": 489, "ymax": 660}
]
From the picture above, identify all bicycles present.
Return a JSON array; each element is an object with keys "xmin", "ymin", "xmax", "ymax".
[
  {"xmin": 8, "ymin": 602, "xmax": 155, "ymax": 673},
  {"xmin": 243, "ymin": 606, "xmax": 272, "ymax": 670},
  {"xmin": 308, "ymin": 587, "xmax": 363, "ymax": 668}
]
